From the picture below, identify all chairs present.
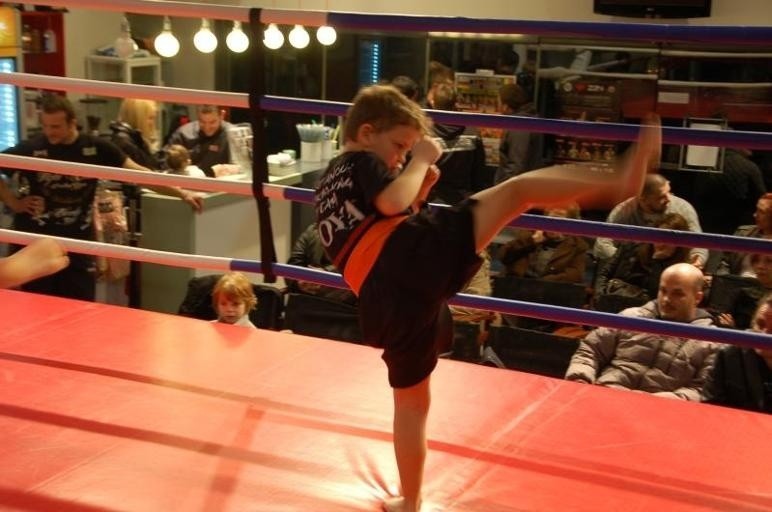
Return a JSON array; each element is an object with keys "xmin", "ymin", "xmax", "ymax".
[
  {"xmin": 444, "ymin": 219, "xmax": 772, "ymax": 409},
  {"xmin": 286, "ymin": 292, "xmax": 363, "ymax": 344},
  {"xmin": 182, "ymin": 275, "xmax": 283, "ymax": 330}
]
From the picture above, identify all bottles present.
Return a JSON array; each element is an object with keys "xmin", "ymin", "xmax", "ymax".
[
  {"xmin": 115, "ymin": 18, "xmax": 139, "ymax": 59},
  {"xmin": 553, "ymin": 137, "xmax": 616, "ymax": 161}
]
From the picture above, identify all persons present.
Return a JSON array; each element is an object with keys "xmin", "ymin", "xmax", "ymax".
[
  {"xmin": 3, "ymin": 87, "xmax": 255, "ymax": 306},
  {"xmin": 207, "ymin": 271, "xmax": 256, "ymax": 325},
  {"xmin": 313, "ymin": 82, "xmax": 659, "ymax": 511},
  {"xmin": 393, "ymin": 62, "xmax": 771, "ymax": 411},
  {"xmin": 284, "ymin": 229, "xmax": 359, "ymax": 344}
]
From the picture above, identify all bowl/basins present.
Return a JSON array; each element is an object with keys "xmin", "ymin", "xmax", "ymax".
[{"xmin": 268, "ymin": 154, "xmax": 298, "ymax": 176}]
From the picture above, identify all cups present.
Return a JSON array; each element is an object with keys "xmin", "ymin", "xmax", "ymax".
[{"xmin": 285, "ymin": 140, "xmax": 338, "ymax": 164}]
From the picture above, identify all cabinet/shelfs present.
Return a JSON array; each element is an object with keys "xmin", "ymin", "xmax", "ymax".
[
  {"xmin": 21, "ymin": 7, "xmax": 66, "ymax": 95},
  {"xmin": 83, "ymin": 54, "xmax": 163, "ymax": 136}
]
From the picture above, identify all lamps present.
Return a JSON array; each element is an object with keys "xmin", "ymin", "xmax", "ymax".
[{"xmin": 115, "ymin": 12, "xmax": 250, "ymax": 60}]
